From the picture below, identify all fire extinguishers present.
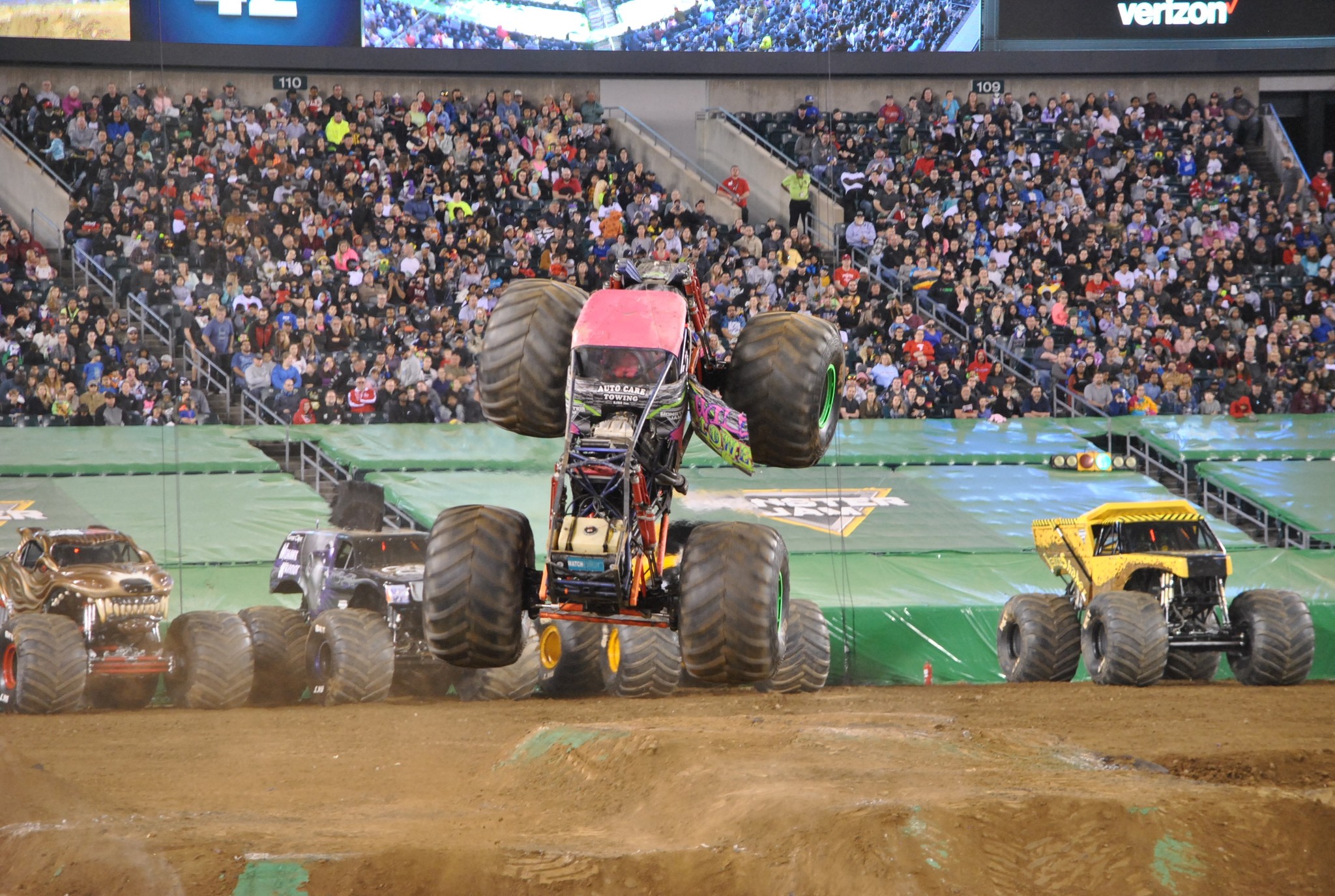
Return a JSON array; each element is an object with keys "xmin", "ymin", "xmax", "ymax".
[{"xmin": 922, "ymin": 660, "xmax": 933, "ymax": 685}]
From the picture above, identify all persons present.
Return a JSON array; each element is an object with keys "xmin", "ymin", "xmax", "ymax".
[
  {"xmin": 364, "ymin": 0, "xmax": 977, "ymax": 54},
  {"xmin": 0, "ymin": 81, "xmax": 1335, "ymax": 423}
]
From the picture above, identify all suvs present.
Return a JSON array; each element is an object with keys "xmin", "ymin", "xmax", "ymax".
[
  {"xmin": 238, "ymin": 530, "xmax": 546, "ymax": 705},
  {"xmin": 996, "ymin": 500, "xmax": 1317, "ymax": 688},
  {"xmin": 424, "ymin": 278, "xmax": 847, "ymax": 688},
  {"xmin": 0, "ymin": 525, "xmax": 255, "ymax": 715}
]
